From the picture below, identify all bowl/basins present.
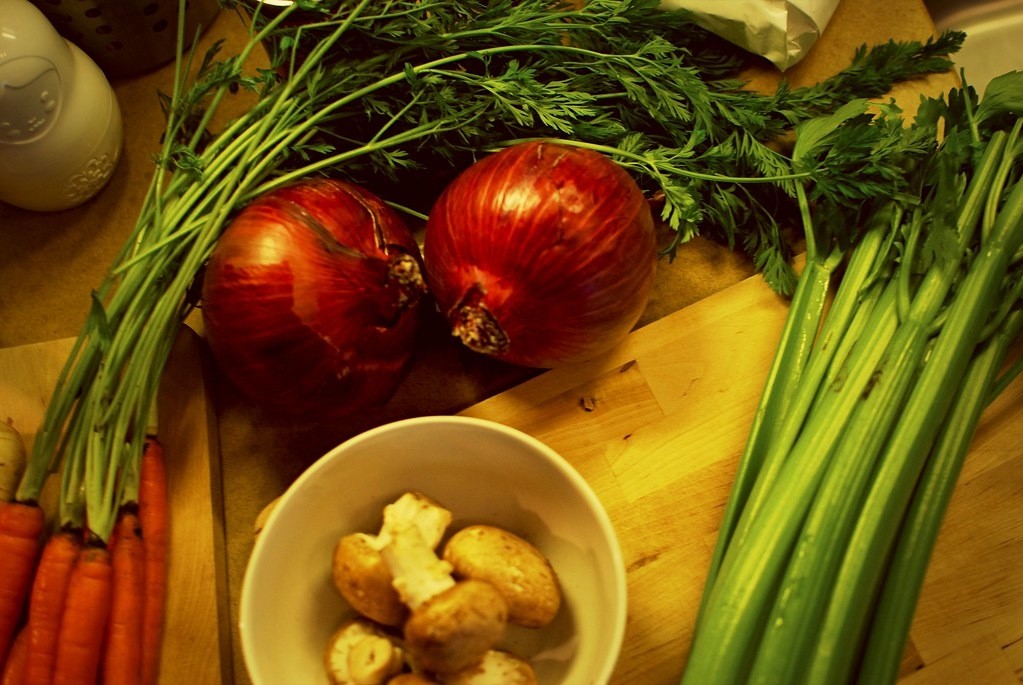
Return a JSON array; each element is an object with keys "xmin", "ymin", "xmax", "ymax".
[{"xmin": 237, "ymin": 414, "xmax": 630, "ymax": 685}]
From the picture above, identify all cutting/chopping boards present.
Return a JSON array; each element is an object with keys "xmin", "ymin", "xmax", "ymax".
[
  {"xmin": 249, "ymin": 191, "xmax": 1022, "ymax": 685},
  {"xmin": 183, "ymin": 4, "xmax": 956, "ymax": 173},
  {"xmin": 1, "ymin": 321, "xmax": 238, "ymax": 685}
]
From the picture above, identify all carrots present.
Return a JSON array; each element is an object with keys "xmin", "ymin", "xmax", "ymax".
[{"xmin": 1, "ymin": 0, "xmax": 970, "ymax": 684}]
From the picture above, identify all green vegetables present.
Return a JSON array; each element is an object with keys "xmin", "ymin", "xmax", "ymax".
[{"xmin": 679, "ymin": 71, "xmax": 1022, "ymax": 681}]
